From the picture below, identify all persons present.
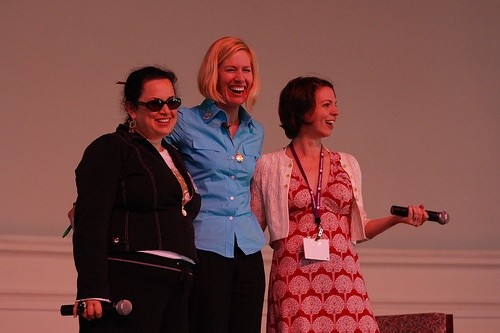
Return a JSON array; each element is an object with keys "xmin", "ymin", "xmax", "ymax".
[
  {"xmin": 72, "ymin": 65, "xmax": 203, "ymax": 333},
  {"xmin": 66, "ymin": 34, "xmax": 268, "ymax": 333},
  {"xmin": 249, "ymin": 75, "xmax": 430, "ymax": 332}
]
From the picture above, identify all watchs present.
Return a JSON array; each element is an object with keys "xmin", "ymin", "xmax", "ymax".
[{"xmin": 76, "ymin": 300, "xmax": 86, "ymax": 315}]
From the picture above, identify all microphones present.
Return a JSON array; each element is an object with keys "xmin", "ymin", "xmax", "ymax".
[
  {"xmin": 390, "ymin": 206, "xmax": 450, "ymax": 225},
  {"xmin": 61, "ymin": 299, "xmax": 132, "ymax": 316},
  {"xmin": 222, "ymin": 122, "xmax": 231, "ymax": 129}
]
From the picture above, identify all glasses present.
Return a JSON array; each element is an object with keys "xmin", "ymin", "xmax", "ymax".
[{"xmin": 135, "ymin": 98, "xmax": 181, "ymax": 112}]
